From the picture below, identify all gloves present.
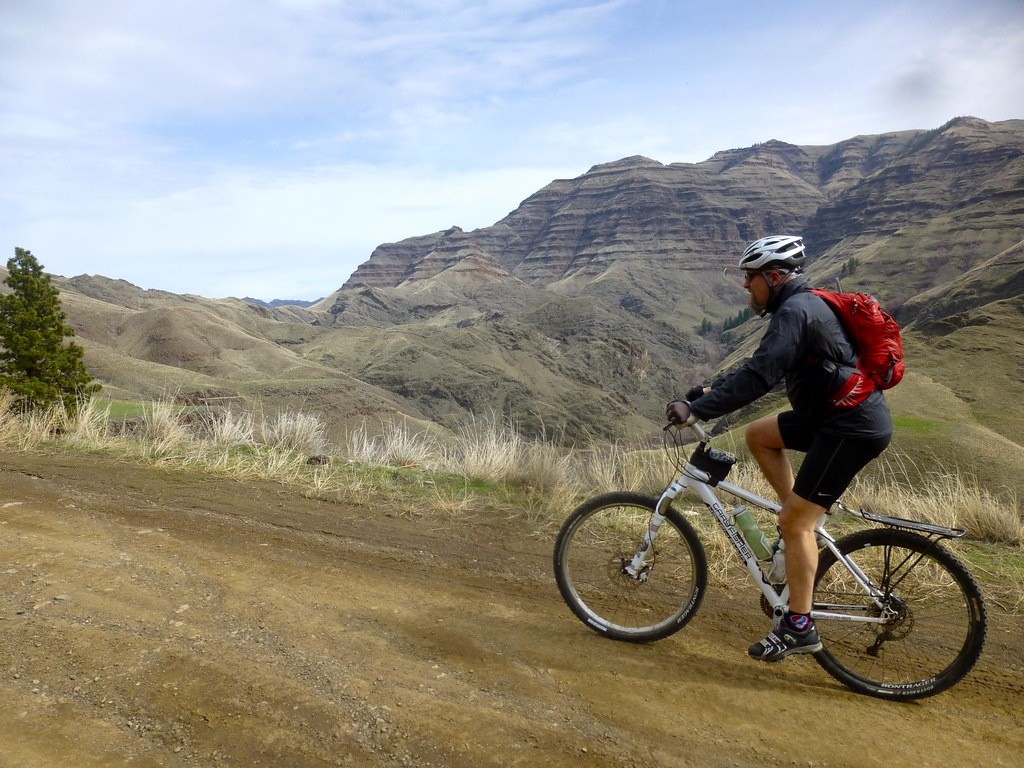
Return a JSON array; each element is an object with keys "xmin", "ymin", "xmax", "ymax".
[
  {"xmin": 684, "ymin": 385, "xmax": 705, "ymax": 402},
  {"xmin": 664, "ymin": 400, "xmax": 698, "ymax": 430}
]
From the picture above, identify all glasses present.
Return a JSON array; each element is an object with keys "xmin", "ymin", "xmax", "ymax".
[{"xmin": 743, "ymin": 271, "xmax": 771, "ymax": 281}]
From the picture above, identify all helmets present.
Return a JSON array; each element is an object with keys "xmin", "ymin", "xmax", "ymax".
[{"xmin": 738, "ymin": 234, "xmax": 807, "ymax": 269}]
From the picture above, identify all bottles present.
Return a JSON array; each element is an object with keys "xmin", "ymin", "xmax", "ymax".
[
  {"xmin": 768, "ymin": 537, "xmax": 787, "ymax": 583},
  {"xmin": 733, "ymin": 506, "xmax": 774, "ymax": 560}
]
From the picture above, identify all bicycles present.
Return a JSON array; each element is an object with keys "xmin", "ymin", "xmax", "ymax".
[{"xmin": 553, "ymin": 398, "xmax": 989, "ymax": 703}]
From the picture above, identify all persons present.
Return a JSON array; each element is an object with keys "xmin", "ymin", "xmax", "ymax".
[{"xmin": 666, "ymin": 235, "xmax": 894, "ymax": 662}]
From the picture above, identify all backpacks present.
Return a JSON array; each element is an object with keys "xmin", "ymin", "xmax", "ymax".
[{"xmin": 806, "ymin": 288, "xmax": 905, "ymax": 410}]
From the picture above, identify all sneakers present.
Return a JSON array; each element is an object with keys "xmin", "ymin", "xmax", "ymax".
[
  {"xmin": 763, "ymin": 524, "xmax": 826, "ymax": 562},
  {"xmin": 747, "ymin": 618, "xmax": 824, "ymax": 662}
]
